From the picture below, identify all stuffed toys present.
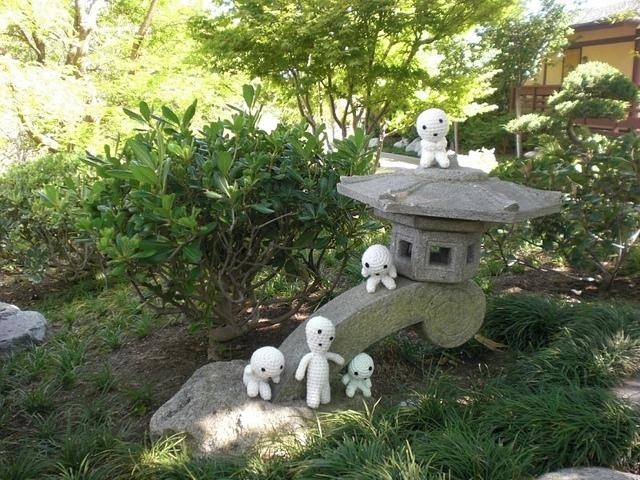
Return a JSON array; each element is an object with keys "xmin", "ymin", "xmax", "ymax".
[
  {"xmin": 360, "ymin": 245, "xmax": 399, "ymax": 293},
  {"xmin": 342, "ymin": 353, "xmax": 374, "ymax": 398},
  {"xmin": 294, "ymin": 315, "xmax": 345, "ymax": 409},
  {"xmin": 416, "ymin": 108, "xmax": 451, "ymax": 169},
  {"xmin": 242, "ymin": 345, "xmax": 286, "ymax": 401}
]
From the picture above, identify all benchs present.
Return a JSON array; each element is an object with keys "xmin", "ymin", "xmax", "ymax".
[{"xmin": 501, "ymin": 81, "xmax": 640, "ymax": 159}]
